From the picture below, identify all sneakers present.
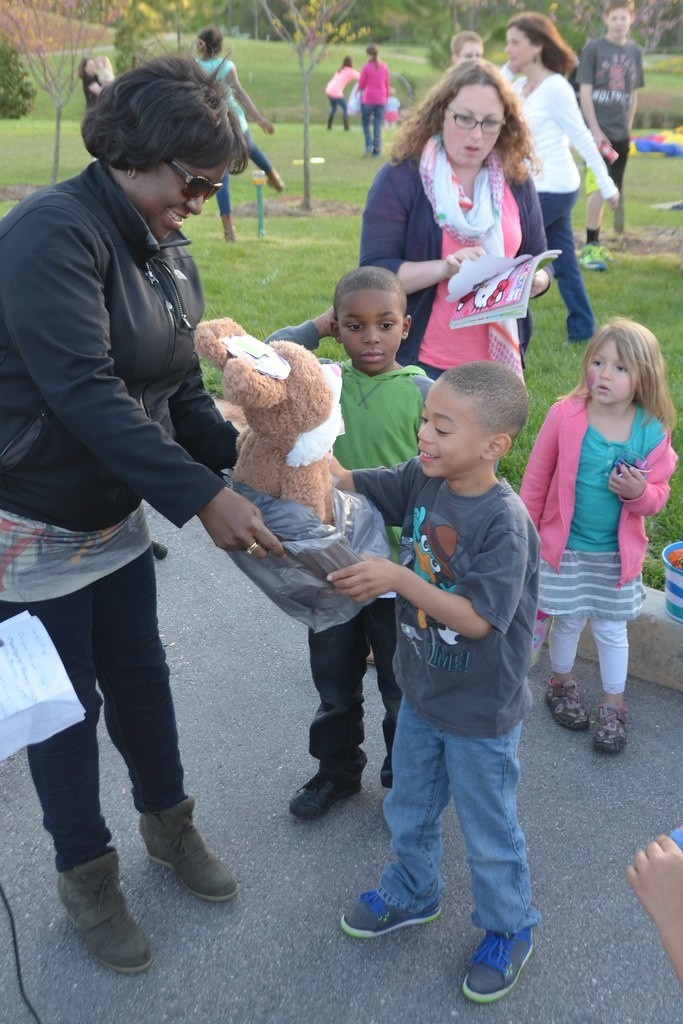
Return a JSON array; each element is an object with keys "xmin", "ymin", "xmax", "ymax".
[
  {"xmin": 289, "ymin": 771, "xmax": 361, "ymax": 820},
  {"xmin": 579, "ymin": 241, "xmax": 608, "ymax": 269},
  {"xmin": 463, "ymin": 927, "xmax": 535, "ymax": 1003},
  {"xmin": 593, "ymin": 701, "xmax": 630, "ymax": 753},
  {"xmin": 545, "ymin": 676, "xmax": 590, "ymax": 730},
  {"xmin": 340, "ymin": 890, "xmax": 440, "ymax": 938},
  {"xmin": 379, "ymin": 756, "xmax": 393, "ymax": 789}
]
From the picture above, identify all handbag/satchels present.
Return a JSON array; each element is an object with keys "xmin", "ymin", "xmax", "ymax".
[{"xmin": 347, "ymin": 83, "xmax": 362, "ymax": 116}]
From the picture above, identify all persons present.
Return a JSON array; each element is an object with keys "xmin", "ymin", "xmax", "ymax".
[
  {"xmin": 497, "ymin": 11, "xmax": 620, "ymax": 347},
  {"xmin": 77, "ymin": 56, "xmax": 115, "ymax": 112},
  {"xmin": 576, "ymin": 0, "xmax": 647, "ymax": 270},
  {"xmin": 264, "ymin": 268, "xmax": 499, "ymax": 819},
  {"xmin": 626, "ymin": 827, "xmax": 683, "ymax": 991},
  {"xmin": 518, "ymin": 317, "xmax": 678, "ymax": 752},
  {"xmin": 325, "ymin": 43, "xmax": 400, "ymax": 158},
  {"xmin": 450, "ymin": 30, "xmax": 485, "ymax": 65},
  {"xmin": 330, "ymin": 360, "xmax": 541, "ymax": 1004},
  {"xmin": 0, "ymin": 54, "xmax": 288, "ymax": 973},
  {"xmin": 191, "ymin": 28, "xmax": 284, "ymax": 243},
  {"xmin": 358, "ymin": 59, "xmax": 555, "ymax": 664}
]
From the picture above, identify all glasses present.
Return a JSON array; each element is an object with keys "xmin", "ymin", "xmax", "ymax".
[
  {"xmin": 163, "ymin": 156, "xmax": 223, "ymax": 203},
  {"xmin": 446, "ymin": 105, "xmax": 507, "ymax": 132}
]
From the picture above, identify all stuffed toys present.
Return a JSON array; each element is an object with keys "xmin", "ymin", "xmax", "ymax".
[{"xmin": 191, "ymin": 316, "xmax": 330, "ymax": 524}]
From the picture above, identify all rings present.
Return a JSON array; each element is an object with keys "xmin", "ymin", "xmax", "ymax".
[{"xmin": 244, "ymin": 543, "xmax": 260, "ymax": 555}]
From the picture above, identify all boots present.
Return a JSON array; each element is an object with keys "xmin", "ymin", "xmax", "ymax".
[
  {"xmin": 138, "ymin": 797, "xmax": 237, "ymax": 900},
  {"xmin": 344, "ymin": 116, "xmax": 350, "ymax": 131},
  {"xmin": 328, "ymin": 115, "xmax": 333, "ymax": 129},
  {"xmin": 267, "ymin": 168, "xmax": 284, "ymax": 192},
  {"xmin": 221, "ymin": 213, "xmax": 237, "ymax": 242},
  {"xmin": 58, "ymin": 847, "xmax": 153, "ymax": 973}
]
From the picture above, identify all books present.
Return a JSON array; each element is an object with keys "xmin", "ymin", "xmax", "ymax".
[{"xmin": 450, "ymin": 250, "xmax": 562, "ymax": 329}]
